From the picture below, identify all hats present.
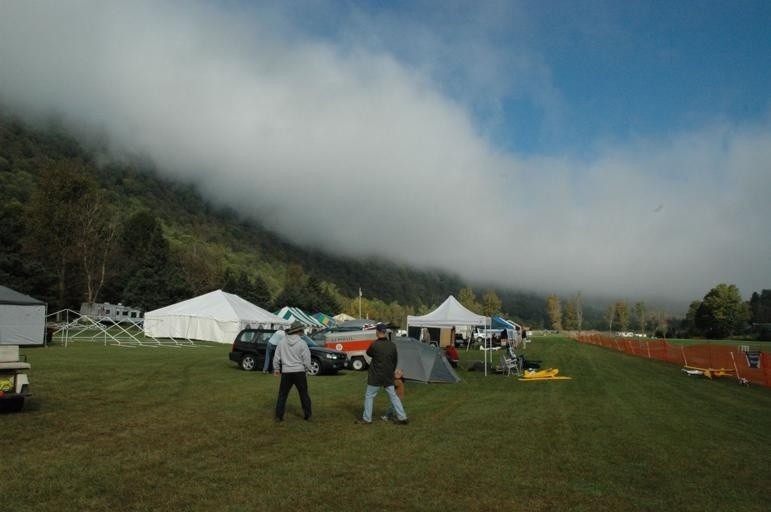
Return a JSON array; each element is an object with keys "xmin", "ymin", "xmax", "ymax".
[
  {"xmin": 377, "ymin": 323, "xmax": 387, "ymax": 331},
  {"xmin": 288, "ymin": 321, "xmax": 306, "ymax": 334}
]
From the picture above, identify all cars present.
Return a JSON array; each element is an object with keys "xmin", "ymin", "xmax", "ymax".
[
  {"xmin": 229, "ymin": 327, "xmax": 407, "ymax": 377},
  {"xmin": 454, "ymin": 327, "xmax": 491, "ymax": 347}
]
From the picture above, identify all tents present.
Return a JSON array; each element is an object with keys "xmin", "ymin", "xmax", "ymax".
[
  {"xmin": 143, "ymin": 289, "xmax": 385, "ymax": 344},
  {"xmin": 392, "ymin": 336, "xmax": 462, "ymax": 384},
  {"xmin": 0, "ymin": 285, "xmax": 48, "ymax": 347}
]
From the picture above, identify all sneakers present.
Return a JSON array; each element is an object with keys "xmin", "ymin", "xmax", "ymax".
[{"xmin": 355, "ymin": 415, "xmax": 409, "ymax": 425}]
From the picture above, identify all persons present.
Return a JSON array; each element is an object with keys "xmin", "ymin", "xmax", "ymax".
[
  {"xmin": 263, "ymin": 329, "xmax": 287, "ymax": 374},
  {"xmin": 522, "ymin": 329, "xmax": 527, "ymax": 350},
  {"xmin": 446, "ymin": 346, "xmax": 460, "ymax": 368},
  {"xmin": 357, "ymin": 324, "xmax": 409, "ymax": 425},
  {"xmin": 380, "ymin": 368, "xmax": 405, "ymax": 422},
  {"xmin": 275, "ymin": 321, "xmax": 313, "ymax": 422}
]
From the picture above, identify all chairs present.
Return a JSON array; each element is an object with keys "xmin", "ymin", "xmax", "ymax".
[{"xmin": 498, "ymin": 354, "xmax": 520, "ymax": 377}]
